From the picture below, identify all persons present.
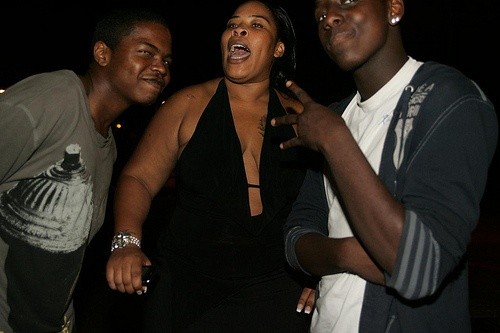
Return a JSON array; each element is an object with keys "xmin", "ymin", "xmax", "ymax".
[
  {"xmin": 106, "ymin": 0, "xmax": 319, "ymax": 332},
  {"xmin": 271, "ymin": 0, "xmax": 500, "ymax": 333},
  {"xmin": 1, "ymin": 0, "xmax": 172, "ymax": 332}
]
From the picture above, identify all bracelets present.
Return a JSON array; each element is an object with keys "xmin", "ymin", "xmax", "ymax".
[{"xmin": 110, "ymin": 232, "xmax": 142, "ymax": 251}]
